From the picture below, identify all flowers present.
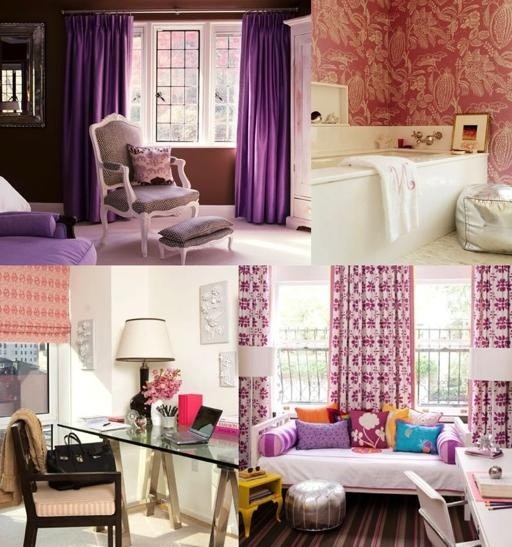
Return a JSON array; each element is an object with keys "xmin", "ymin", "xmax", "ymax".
[{"xmin": 143, "ymin": 367, "xmax": 184, "ymax": 407}]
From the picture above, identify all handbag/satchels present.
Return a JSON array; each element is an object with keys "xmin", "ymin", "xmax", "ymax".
[{"xmin": 44, "ymin": 431, "xmax": 118, "ymax": 492}]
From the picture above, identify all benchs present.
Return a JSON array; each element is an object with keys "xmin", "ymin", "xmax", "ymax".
[{"xmin": 248, "ymin": 410, "xmax": 473, "ymax": 525}]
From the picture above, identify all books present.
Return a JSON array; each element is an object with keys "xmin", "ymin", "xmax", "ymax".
[
  {"xmin": 239, "ymin": 475, "xmax": 267, "ymax": 482},
  {"xmin": 88, "ymin": 418, "xmax": 128, "ymax": 432},
  {"xmin": 250, "ymin": 490, "xmax": 272, "ymax": 502},
  {"xmin": 472, "ymin": 471, "xmax": 512, "ymax": 499}
]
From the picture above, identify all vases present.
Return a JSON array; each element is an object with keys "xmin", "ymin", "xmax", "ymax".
[{"xmin": 150, "ymin": 394, "xmax": 179, "ymax": 426}]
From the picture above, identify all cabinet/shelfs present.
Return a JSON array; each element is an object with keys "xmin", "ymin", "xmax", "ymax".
[{"xmin": 283, "ymin": 13, "xmax": 314, "ymax": 233}]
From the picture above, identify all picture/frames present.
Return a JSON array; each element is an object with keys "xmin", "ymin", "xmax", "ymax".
[{"xmin": 451, "ymin": 111, "xmax": 490, "ymax": 153}]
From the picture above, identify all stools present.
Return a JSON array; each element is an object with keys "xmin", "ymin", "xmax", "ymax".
[
  {"xmin": 157, "ymin": 227, "xmax": 236, "ymax": 265},
  {"xmin": 456, "ymin": 182, "xmax": 512, "ymax": 255},
  {"xmin": 285, "ymin": 479, "xmax": 348, "ymax": 532}
]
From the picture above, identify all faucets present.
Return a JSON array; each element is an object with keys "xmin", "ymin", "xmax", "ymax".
[{"xmin": 416, "ymin": 136, "xmax": 434, "ymax": 145}]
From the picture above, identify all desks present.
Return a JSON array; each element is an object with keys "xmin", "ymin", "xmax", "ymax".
[
  {"xmin": 453, "ymin": 445, "xmax": 511, "ymax": 546},
  {"xmin": 239, "ymin": 473, "xmax": 284, "ymax": 540},
  {"xmin": 55, "ymin": 414, "xmax": 240, "ymax": 547}
]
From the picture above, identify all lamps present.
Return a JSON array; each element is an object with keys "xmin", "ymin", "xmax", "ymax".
[
  {"xmin": 116, "ymin": 317, "xmax": 177, "ymax": 417},
  {"xmin": 468, "ymin": 346, "xmax": 512, "ymax": 448},
  {"xmin": 239, "ymin": 344, "xmax": 271, "ymax": 426}
]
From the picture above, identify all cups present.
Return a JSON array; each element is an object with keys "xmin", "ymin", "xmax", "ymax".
[
  {"xmin": 398, "ymin": 138, "xmax": 404, "ymax": 148},
  {"xmin": 162, "ymin": 416, "xmax": 177, "ymax": 428}
]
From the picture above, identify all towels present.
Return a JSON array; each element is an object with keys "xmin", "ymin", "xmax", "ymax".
[{"xmin": 340, "ymin": 153, "xmax": 420, "ymax": 243}]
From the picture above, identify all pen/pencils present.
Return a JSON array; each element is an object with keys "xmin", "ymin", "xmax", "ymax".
[
  {"xmin": 103, "ymin": 423, "xmax": 110, "ymax": 426},
  {"xmin": 156, "ymin": 404, "xmax": 178, "ymax": 417},
  {"xmin": 484, "ymin": 500, "xmax": 512, "ymax": 511}
]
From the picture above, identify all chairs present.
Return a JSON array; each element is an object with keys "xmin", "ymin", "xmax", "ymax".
[
  {"xmin": 1, "ymin": 211, "xmax": 96, "ymax": 265},
  {"xmin": 9, "ymin": 418, "xmax": 124, "ymax": 547},
  {"xmin": 89, "ymin": 111, "xmax": 202, "ymax": 259},
  {"xmin": 404, "ymin": 467, "xmax": 482, "ymax": 546}
]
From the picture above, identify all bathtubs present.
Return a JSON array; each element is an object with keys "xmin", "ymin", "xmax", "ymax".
[{"xmin": 311, "ymin": 147, "xmax": 491, "ymax": 250}]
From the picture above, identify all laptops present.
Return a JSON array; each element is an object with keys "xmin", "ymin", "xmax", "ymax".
[{"xmin": 162, "ymin": 405, "xmax": 224, "ymax": 444}]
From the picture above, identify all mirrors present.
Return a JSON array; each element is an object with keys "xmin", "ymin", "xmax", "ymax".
[{"xmin": 0, "ymin": 21, "xmax": 49, "ymax": 129}]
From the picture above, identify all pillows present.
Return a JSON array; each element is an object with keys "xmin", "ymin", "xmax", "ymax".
[
  {"xmin": 1, "ymin": 176, "xmax": 33, "ymax": 213},
  {"xmin": 437, "ymin": 423, "xmax": 463, "ymax": 464},
  {"xmin": 327, "ymin": 406, "xmax": 349, "ymax": 423},
  {"xmin": 405, "ymin": 406, "xmax": 445, "ymax": 429},
  {"xmin": 294, "ymin": 419, "xmax": 351, "ymax": 452},
  {"xmin": 383, "ymin": 403, "xmax": 411, "ymax": 446},
  {"xmin": 393, "ymin": 418, "xmax": 445, "ymax": 457},
  {"xmin": 295, "ymin": 403, "xmax": 338, "ymax": 426},
  {"xmin": 348, "ymin": 409, "xmax": 390, "ymax": 449},
  {"xmin": 156, "ymin": 211, "xmax": 231, "ymax": 241},
  {"xmin": 258, "ymin": 420, "xmax": 298, "ymax": 456},
  {"xmin": 125, "ymin": 142, "xmax": 175, "ymax": 185}
]
